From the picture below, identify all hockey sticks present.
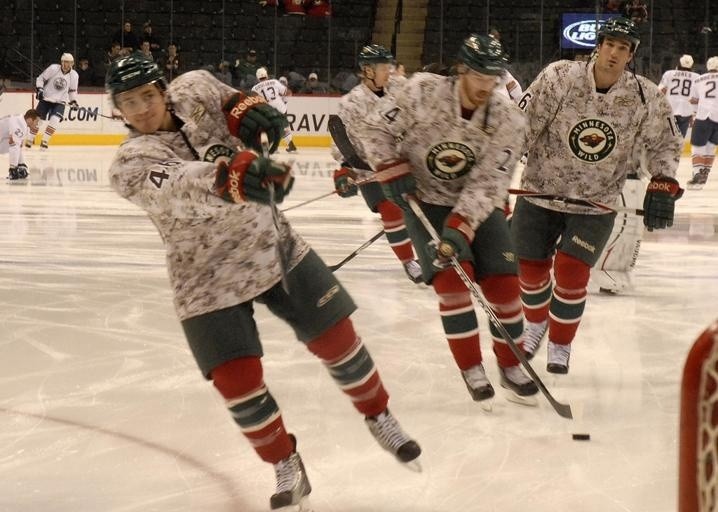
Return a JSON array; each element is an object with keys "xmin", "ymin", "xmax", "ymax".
[
  {"xmin": 408, "ymin": 199, "xmax": 595, "ymax": 418},
  {"xmin": 261, "ymin": 131, "xmax": 291, "ymax": 295},
  {"xmin": 327, "ymin": 231, "xmax": 385, "ymax": 272},
  {"xmin": 328, "ymin": 114, "xmax": 645, "ymax": 217},
  {"xmin": 76, "ymin": 107, "xmax": 124, "ymax": 120}
]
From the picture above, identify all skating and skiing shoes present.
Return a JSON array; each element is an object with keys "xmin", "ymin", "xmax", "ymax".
[{"xmin": 25, "ymin": 140, "xmax": 48, "ymax": 148}]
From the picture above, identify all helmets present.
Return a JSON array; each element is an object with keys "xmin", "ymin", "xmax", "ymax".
[
  {"xmin": 706, "ymin": 56, "xmax": 718, "ymax": 71},
  {"xmin": 358, "ymin": 45, "xmax": 392, "ymax": 63},
  {"xmin": 106, "ymin": 56, "xmax": 167, "ymax": 94},
  {"xmin": 256, "ymin": 68, "xmax": 268, "ymax": 80},
  {"xmin": 458, "ymin": 33, "xmax": 505, "ymax": 73},
  {"xmin": 309, "ymin": 73, "xmax": 318, "ymax": 80},
  {"xmin": 598, "ymin": 15, "xmax": 639, "ymax": 52},
  {"xmin": 61, "ymin": 52, "xmax": 74, "ymax": 62},
  {"xmin": 680, "ymin": 54, "xmax": 694, "ymax": 68},
  {"xmin": 279, "ymin": 76, "xmax": 288, "ymax": 84}
]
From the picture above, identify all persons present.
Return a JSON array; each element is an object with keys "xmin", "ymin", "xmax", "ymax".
[
  {"xmin": 106, "ymin": 53, "xmax": 422, "ymax": 509},
  {"xmin": 444, "ymin": 64, "xmax": 522, "ymax": 233},
  {"xmin": 518, "ymin": 16, "xmax": 683, "ymax": 375},
  {"xmin": 0, "ymin": 108, "xmax": 39, "ymax": 180},
  {"xmin": 691, "ymin": 55, "xmax": 718, "ymax": 184},
  {"xmin": 25, "ymin": 51, "xmax": 78, "ymax": 146},
  {"xmin": 79, "ymin": 55, "xmax": 94, "ymax": 86},
  {"xmin": 329, "ymin": 45, "xmax": 424, "ymax": 284},
  {"xmin": 360, "ymin": 31, "xmax": 539, "ymax": 404},
  {"xmin": 100, "ymin": 20, "xmax": 184, "ymax": 84},
  {"xmin": 198, "ymin": 47, "xmax": 335, "ymax": 94},
  {"xmin": 657, "ymin": 52, "xmax": 695, "ymax": 139}
]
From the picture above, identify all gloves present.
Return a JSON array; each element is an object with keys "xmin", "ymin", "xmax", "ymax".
[
  {"xmin": 216, "ymin": 152, "xmax": 293, "ymax": 203},
  {"xmin": 9, "ymin": 164, "xmax": 27, "ymax": 179},
  {"xmin": 223, "ymin": 90, "xmax": 288, "ymax": 153},
  {"xmin": 36, "ymin": 87, "xmax": 44, "ymax": 100},
  {"xmin": 70, "ymin": 101, "xmax": 78, "ymax": 110},
  {"xmin": 335, "ymin": 168, "xmax": 359, "ymax": 197},
  {"xmin": 378, "ymin": 157, "xmax": 415, "ymax": 209},
  {"xmin": 426, "ymin": 213, "xmax": 476, "ymax": 271},
  {"xmin": 643, "ymin": 174, "xmax": 679, "ymax": 232}
]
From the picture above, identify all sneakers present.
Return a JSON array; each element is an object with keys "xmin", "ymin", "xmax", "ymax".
[
  {"xmin": 405, "ymin": 260, "xmax": 424, "ymax": 282},
  {"xmin": 365, "ymin": 408, "xmax": 420, "ymax": 461},
  {"xmin": 523, "ymin": 321, "xmax": 571, "ymax": 374},
  {"xmin": 270, "ymin": 452, "xmax": 311, "ymax": 509},
  {"xmin": 462, "ymin": 364, "xmax": 539, "ymax": 400},
  {"xmin": 689, "ymin": 176, "xmax": 706, "ymax": 184}
]
